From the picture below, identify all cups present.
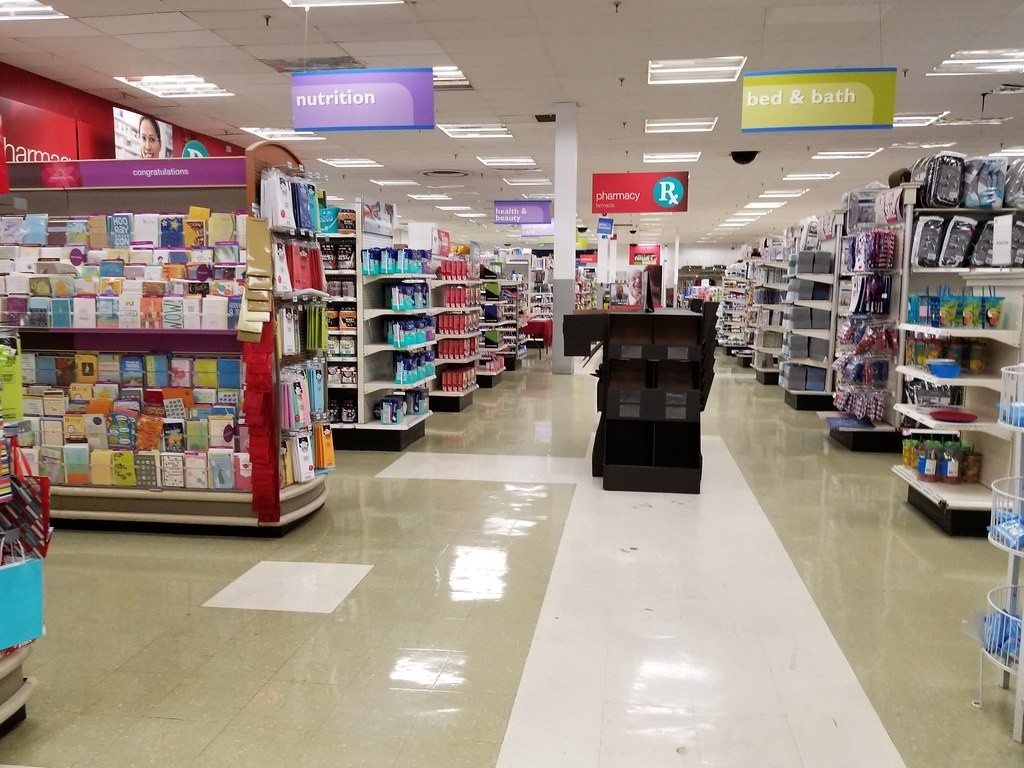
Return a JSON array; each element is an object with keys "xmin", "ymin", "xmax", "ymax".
[
  {"xmin": 906, "ymin": 284, "xmax": 1007, "ymax": 328},
  {"xmin": 905, "ymin": 332, "xmax": 988, "ymax": 375},
  {"xmin": 901, "ymin": 432, "xmax": 983, "ymax": 485}
]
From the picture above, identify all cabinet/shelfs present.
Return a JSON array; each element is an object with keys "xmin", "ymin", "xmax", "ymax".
[{"xmin": 0, "ymin": 155, "xmax": 1023, "ymax": 746}]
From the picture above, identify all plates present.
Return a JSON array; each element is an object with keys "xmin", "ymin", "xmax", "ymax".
[{"xmin": 928, "ymin": 410, "xmax": 977, "ymax": 422}]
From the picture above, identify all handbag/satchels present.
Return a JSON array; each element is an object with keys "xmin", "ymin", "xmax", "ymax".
[
  {"xmin": 0, "ymin": 437, "xmax": 50, "ymax": 558},
  {"xmin": 0, "ymin": 536, "xmax": 43, "ymax": 651}
]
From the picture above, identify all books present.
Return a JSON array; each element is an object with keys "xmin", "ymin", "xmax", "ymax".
[{"xmin": 0, "ymin": 207, "xmax": 254, "ymax": 492}]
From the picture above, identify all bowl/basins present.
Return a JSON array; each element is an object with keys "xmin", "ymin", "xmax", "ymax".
[{"xmin": 926, "ymin": 358, "xmax": 961, "ymax": 379}]
